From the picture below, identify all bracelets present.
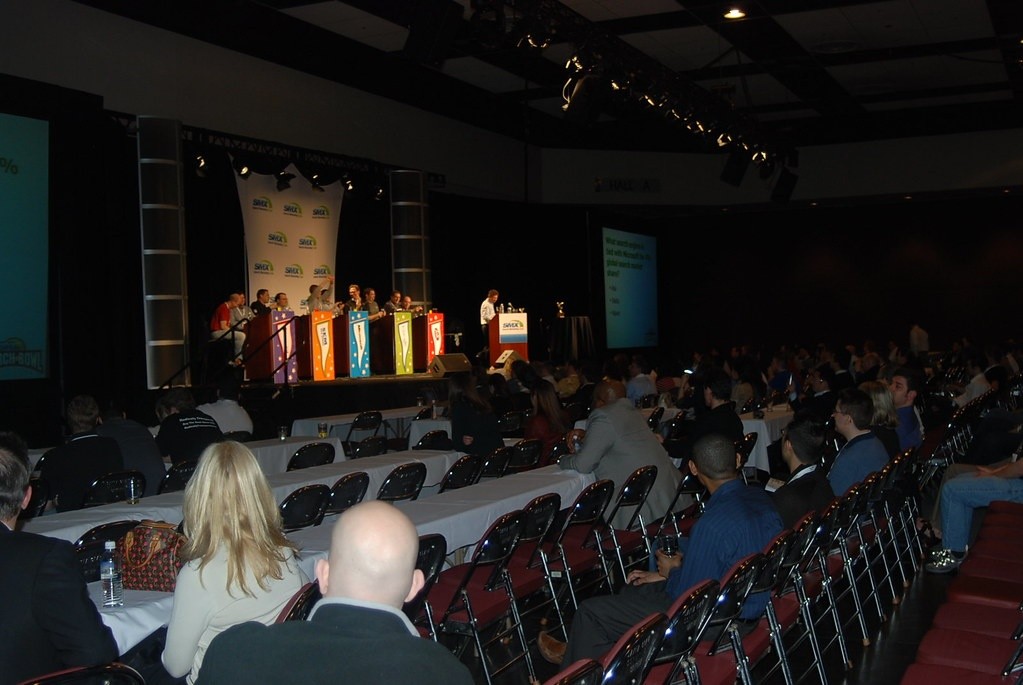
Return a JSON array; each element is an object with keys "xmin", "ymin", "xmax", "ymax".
[{"xmin": 330, "ymin": 282, "xmax": 334, "ymax": 284}]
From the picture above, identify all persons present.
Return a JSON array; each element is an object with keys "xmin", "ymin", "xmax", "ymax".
[
  {"xmin": 209, "ymin": 293, "xmax": 246, "ymax": 368},
  {"xmin": 159, "ymin": 442, "xmax": 304, "ymax": 685},
  {"xmin": 0, "ymin": 432, "xmax": 119, "ymax": 685},
  {"xmin": 186, "ymin": 499, "xmax": 476, "ymax": 685},
  {"xmin": 231, "ymin": 273, "xmax": 423, "ymax": 327},
  {"xmin": 449, "ymin": 319, "xmax": 1023, "ymax": 574},
  {"xmin": 152, "ymin": 389, "xmax": 225, "ymax": 494},
  {"xmin": 475, "ymin": 289, "xmax": 498, "ymax": 366},
  {"xmin": 537, "ymin": 431, "xmax": 786, "ymax": 684},
  {"xmin": 95, "ymin": 395, "xmax": 166, "ymax": 502},
  {"xmin": 30, "ymin": 394, "xmax": 121, "ymax": 514},
  {"xmin": 195, "ymin": 377, "xmax": 253, "ymax": 434}
]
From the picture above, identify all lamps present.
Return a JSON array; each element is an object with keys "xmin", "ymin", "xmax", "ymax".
[
  {"xmin": 187, "ymin": 141, "xmax": 385, "ymax": 197},
  {"xmin": 466, "ymin": 0, "xmax": 784, "ymax": 181}
]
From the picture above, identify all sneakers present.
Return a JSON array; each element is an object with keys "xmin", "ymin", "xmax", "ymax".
[{"xmin": 927, "ymin": 544, "xmax": 970, "ymax": 569}]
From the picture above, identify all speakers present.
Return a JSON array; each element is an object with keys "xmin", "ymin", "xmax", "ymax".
[{"xmin": 428, "ymin": 353, "xmax": 473, "ymax": 378}]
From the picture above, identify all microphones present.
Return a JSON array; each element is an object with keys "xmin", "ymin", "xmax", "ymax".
[{"xmin": 508, "ymin": 303, "xmax": 515, "ymax": 310}]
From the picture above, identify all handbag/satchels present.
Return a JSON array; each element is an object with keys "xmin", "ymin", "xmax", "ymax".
[{"xmin": 114, "ymin": 517, "xmax": 185, "ymax": 594}]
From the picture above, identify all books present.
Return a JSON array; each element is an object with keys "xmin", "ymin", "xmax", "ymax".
[
  {"xmin": 772, "ymin": 403, "xmax": 790, "ymax": 411},
  {"xmin": 765, "ymin": 478, "xmax": 785, "ymax": 493}
]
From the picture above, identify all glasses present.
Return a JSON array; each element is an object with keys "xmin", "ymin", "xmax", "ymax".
[{"xmin": 832, "ymin": 408, "xmax": 849, "ymax": 414}]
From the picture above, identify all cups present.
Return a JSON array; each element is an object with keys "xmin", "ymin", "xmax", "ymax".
[
  {"xmin": 278, "ymin": 426, "xmax": 288, "ymax": 441},
  {"xmin": 318, "ymin": 423, "xmax": 327, "ymax": 439},
  {"xmin": 417, "ymin": 397, "xmax": 423, "ymax": 409},
  {"xmin": 659, "ymin": 536, "xmax": 677, "ymax": 555}
]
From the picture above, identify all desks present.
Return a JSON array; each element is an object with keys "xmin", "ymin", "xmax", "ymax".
[
  {"xmin": 574, "ymin": 406, "xmax": 682, "ymax": 450},
  {"xmin": 87, "ymin": 550, "xmax": 326, "ymax": 658},
  {"xmin": 525, "ymin": 464, "xmax": 596, "ymax": 489},
  {"xmin": 408, "ymin": 416, "xmax": 453, "ymax": 449},
  {"xmin": 17, "ymin": 489, "xmax": 184, "ymax": 543},
  {"xmin": 243, "ymin": 436, "xmax": 346, "ymax": 475},
  {"xmin": 291, "ymin": 405, "xmax": 426, "ymax": 440},
  {"xmin": 543, "ymin": 315, "xmax": 596, "ymax": 362},
  {"xmin": 264, "ymin": 449, "xmax": 470, "ymax": 504},
  {"xmin": 283, "ymin": 473, "xmax": 584, "ymax": 588},
  {"xmin": 737, "ymin": 403, "xmax": 796, "ymax": 485}
]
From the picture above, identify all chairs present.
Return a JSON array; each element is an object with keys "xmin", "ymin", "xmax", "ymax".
[
  {"xmin": 73, "ymin": 515, "xmax": 140, "ymax": 582},
  {"xmin": 9, "ymin": 355, "xmax": 1023, "ymax": 685}
]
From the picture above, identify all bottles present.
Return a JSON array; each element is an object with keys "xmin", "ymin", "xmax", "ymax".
[
  {"xmin": 100, "ymin": 541, "xmax": 123, "ymax": 608},
  {"xmin": 573, "ymin": 435, "xmax": 582, "ymax": 454},
  {"xmin": 431, "ymin": 400, "xmax": 437, "ymax": 419},
  {"xmin": 127, "ymin": 469, "xmax": 139, "ymax": 504},
  {"xmin": 658, "ymin": 400, "xmax": 667, "ymax": 410},
  {"xmin": 500, "ymin": 304, "xmax": 504, "ymax": 313}
]
